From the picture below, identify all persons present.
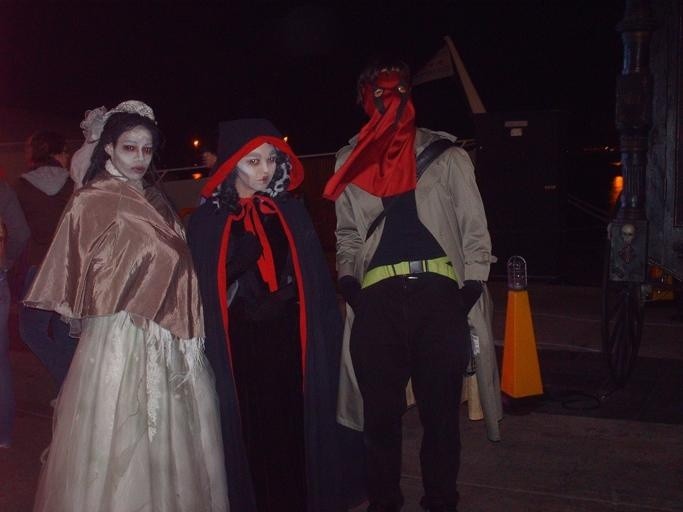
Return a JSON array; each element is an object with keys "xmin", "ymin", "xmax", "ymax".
[
  {"xmin": 203, "ymin": 146, "xmax": 222, "ymax": 177},
  {"xmin": 0, "ymin": 125, "xmax": 79, "ymax": 448},
  {"xmin": 334, "ymin": 54, "xmax": 506, "ymax": 510},
  {"xmin": 19, "ymin": 98, "xmax": 228, "ymax": 512},
  {"xmin": 182, "ymin": 118, "xmax": 364, "ymax": 512}
]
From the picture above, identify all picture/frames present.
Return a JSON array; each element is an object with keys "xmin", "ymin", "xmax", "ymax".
[{"xmin": 607, "ymin": 214, "xmax": 651, "ymax": 283}]
